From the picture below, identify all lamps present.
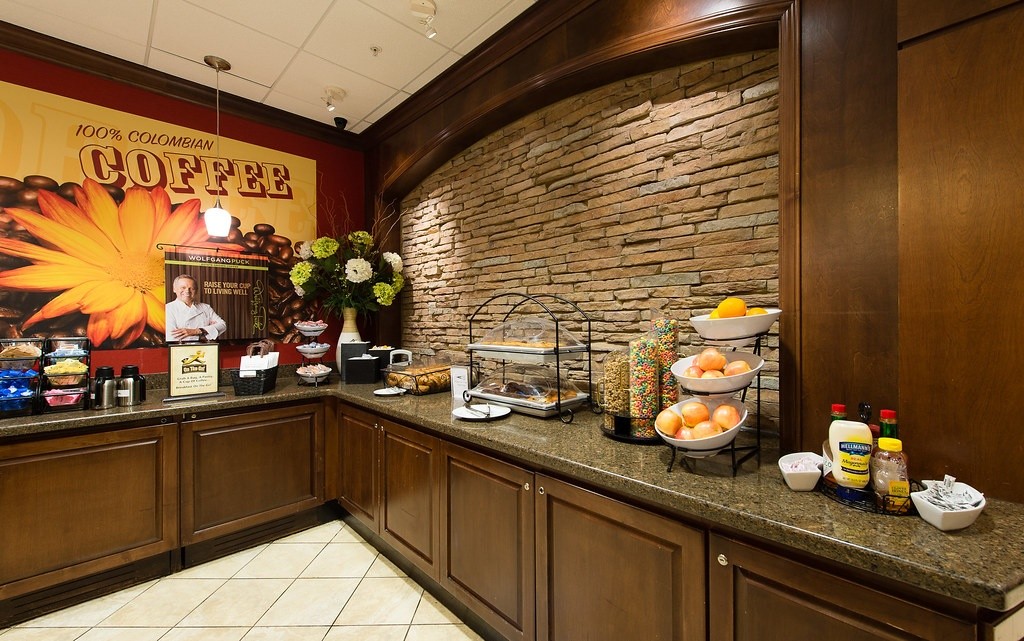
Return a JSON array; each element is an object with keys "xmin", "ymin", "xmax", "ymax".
[
  {"xmin": 320, "ymin": 86, "xmax": 347, "ymax": 110},
  {"xmin": 203, "ymin": 55, "xmax": 232, "ymax": 237},
  {"xmin": 408, "ymin": 0, "xmax": 439, "ymax": 39}
]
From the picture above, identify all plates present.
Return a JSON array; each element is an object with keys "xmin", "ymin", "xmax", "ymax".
[
  {"xmin": 373, "ymin": 387, "xmax": 408, "ymax": 396},
  {"xmin": 452, "ymin": 403, "xmax": 511, "ymax": 420}
]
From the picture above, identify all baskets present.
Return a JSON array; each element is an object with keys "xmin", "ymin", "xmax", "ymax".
[
  {"xmin": 818, "ymin": 475, "xmax": 925, "ymax": 516},
  {"xmin": 231, "ymin": 365, "xmax": 278, "ymax": 396}
]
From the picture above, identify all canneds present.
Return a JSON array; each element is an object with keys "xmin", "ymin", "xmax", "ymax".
[{"xmin": 869, "ymin": 437, "xmax": 909, "ymax": 513}]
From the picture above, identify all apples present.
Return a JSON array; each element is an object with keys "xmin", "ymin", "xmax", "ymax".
[
  {"xmin": 655, "ymin": 402, "xmax": 740, "ymax": 440},
  {"xmin": 682, "ymin": 347, "xmax": 752, "ymax": 378}
]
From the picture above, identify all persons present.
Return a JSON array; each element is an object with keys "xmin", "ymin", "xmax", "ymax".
[{"xmin": 166, "ymin": 275, "xmax": 227, "ymax": 342}]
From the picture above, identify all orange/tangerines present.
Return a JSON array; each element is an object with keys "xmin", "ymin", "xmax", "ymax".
[{"xmin": 707, "ymin": 297, "xmax": 768, "ymax": 319}]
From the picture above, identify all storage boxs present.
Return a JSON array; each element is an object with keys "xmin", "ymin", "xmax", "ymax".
[
  {"xmin": 366, "ymin": 347, "xmax": 395, "ymax": 375},
  {"xmin": 339, "ymin": 341, "xmax": 370, "ymax": 382},
  {"xmin": 345, "ymin": 357, "xmax": 380, "ymax": 386}
]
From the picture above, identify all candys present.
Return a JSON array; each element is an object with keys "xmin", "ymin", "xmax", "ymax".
[
  {"xmin": 604, "ymin": 319, "xmax": 679, "ymax": 419},
  {"xmin": 0, "ymin": 344, "xmax": 89, "ymax": 412},
  {"xmin": 295, "ymin": 320, "xmax": 329, "ymax": 374},
  {"xmin": 630, "ymin": 418, "xmax": 655, "ymax": 438}
]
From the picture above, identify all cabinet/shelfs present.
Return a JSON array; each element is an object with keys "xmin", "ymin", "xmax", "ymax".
[
  {"xmin": 463, "ymin": 294, "xmax": 593, "ymax": 419},
  {"xmin": 445, "ymin": 439, "xmax": 707, "ymax": 641},
  {"xmin": 0, "ymin": 335, "xmax": 90, "ymax": 417},
  {"xmin": 179, "ymin": 404, "xmax": 325, "ymax": 548},
  {"xmin": 0, "ymin": 422, "xmax": 179, "ymax": 603},
  {"xmin": 706, "ymin": 533, "xmax": 991, "ymax": 640},
  {"xmin": 332, "ymin": 400, "xmax": 442, "ymax": 588}
]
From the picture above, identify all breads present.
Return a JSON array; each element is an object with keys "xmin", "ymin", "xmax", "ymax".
[
  {"xmin": 386, "ymin": 365, "xmax": 451, "ymax": 394},
  {"xmin": 480, "ymin": 381, "xmax": 576, "ymax": 404},
  {"xmin": 483, "ymin": 339, "xmax": 567, "ymax": 348}
]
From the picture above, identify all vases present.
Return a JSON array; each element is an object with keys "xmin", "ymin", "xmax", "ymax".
[{"xmin": 336, "ymin": 308, "xmax": 364, "ymax": 373}]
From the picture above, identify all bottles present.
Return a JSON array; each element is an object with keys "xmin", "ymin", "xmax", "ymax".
[
  {"xmin": 118, "ymin": 366, "xmax": 146, "ymax": 406},
  {"xmin": 89, "ymin": 367, "xmax": 116, "ymax": 409},
  {"xmin": 870, "ymin": 437, "xmax": 909, "ymax": 514},
  {"xmin": 829, "ymin": 420, "xmax": 872, "ymax": 500},
  {"xmin": 830, "ymin": 404, "xmax": 897, "ymax": 438}
]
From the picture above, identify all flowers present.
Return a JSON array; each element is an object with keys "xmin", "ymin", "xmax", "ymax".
[{"xmin": 290, "ymin": 231, "xmax": 403, "ymax": 304}]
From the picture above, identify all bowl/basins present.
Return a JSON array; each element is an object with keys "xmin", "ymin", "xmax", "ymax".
[
  {"xmin": 296, "ymin": 344, "xmax": 331, "ymax": 359},
  {"xmin": 296, "ymin": 365, "xmax": 332, "ymax": 384},
  {"xmin": 778, "ymin": 451, "xmax": 823, "ymax": 491},
  {"xmin": 294, "ymin": 322, "xmax": 328, "ymax": 336},
  {"xmin": 689, "ymin": 308, "xmax": 783, "ymax": 348},
  {"xmin": 910, "ymin": 479, "xmax": 985, "ymax": 531},
  {"xmin": 0, "ymin": 338, "xmax": 88, "ymax": 411},
  {"xmin": 671, "ymin": 351, "xmax": 764, "ymax": 394},
  {"xmin": 655, "ymin": 397, "xmax": 747, "ymax": 458}
]
